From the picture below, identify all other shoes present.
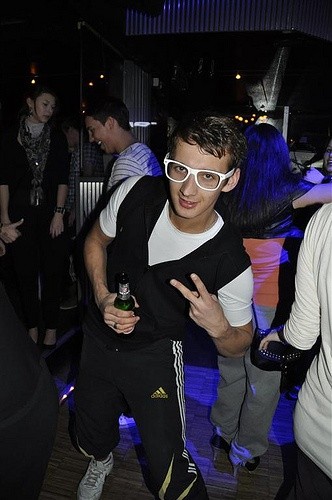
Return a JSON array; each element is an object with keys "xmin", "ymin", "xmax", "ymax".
[
  {"xmin": 43, "ymin": 342, "xmax": 56, "ymax": 351},
  {"xmin": 60, "ymin": 299, "xmax": 77, "ymax": 310}
]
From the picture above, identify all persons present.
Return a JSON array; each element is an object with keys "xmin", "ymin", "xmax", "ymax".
[
  {"xmin": 259, "ymin": 202, "xmax": 332, "ymax": 500},
  {"xmin": 83, "ymin": 98, "xmax": 163, "ymax": 193},
  {"xmin": 208, "ymin": 123, "xmax": 332, "ymax": 475},
  {"xmin": 0, "ymin": 85, "xmax": 103, "ymax": 351},
  {"xmin": 68, "ymin": 116, "xmax": 254, "ymax": 500}
]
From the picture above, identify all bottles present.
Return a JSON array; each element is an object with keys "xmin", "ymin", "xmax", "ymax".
[{"xmin": 113, "ymin": 271, "xmax": 135, "ymax": 335}]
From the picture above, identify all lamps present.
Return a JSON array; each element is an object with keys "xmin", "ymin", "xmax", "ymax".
[{"xmin": 170, "ymin": 56, "xmax": 218, "ymax": 94}]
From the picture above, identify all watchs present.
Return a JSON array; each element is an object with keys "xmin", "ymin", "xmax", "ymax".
[{"xmin": 55, "ymin": 207, "xmax": 66, "ymax": 214}]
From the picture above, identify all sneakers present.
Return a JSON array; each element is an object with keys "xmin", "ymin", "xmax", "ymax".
[{"xmin": 77, "ymin": 452, "xmax": 114, "ymax": 500}]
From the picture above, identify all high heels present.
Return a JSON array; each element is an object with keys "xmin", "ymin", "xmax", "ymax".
[
  {"xmin": 234, "ymin": 456, "xmax": 260, "ymax": 477},
  {"xmin": 210, "ymin": 433, "xmax": 231, "ymax": 461}
]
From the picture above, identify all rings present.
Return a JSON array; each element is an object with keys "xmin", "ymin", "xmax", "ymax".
[{"xmin": 114, "ymin": 322, "xmax": 117, "ymax": 329}]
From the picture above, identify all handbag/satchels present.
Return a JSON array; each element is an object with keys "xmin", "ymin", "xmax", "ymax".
[{"xmin": 250, "ymin": 328, "xmax": 302, "ymax": 372}]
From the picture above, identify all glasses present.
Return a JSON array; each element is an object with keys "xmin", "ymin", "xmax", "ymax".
[{"xmin": 163, "ymin": 151, "xmax": 235, "ymax": 191}]
